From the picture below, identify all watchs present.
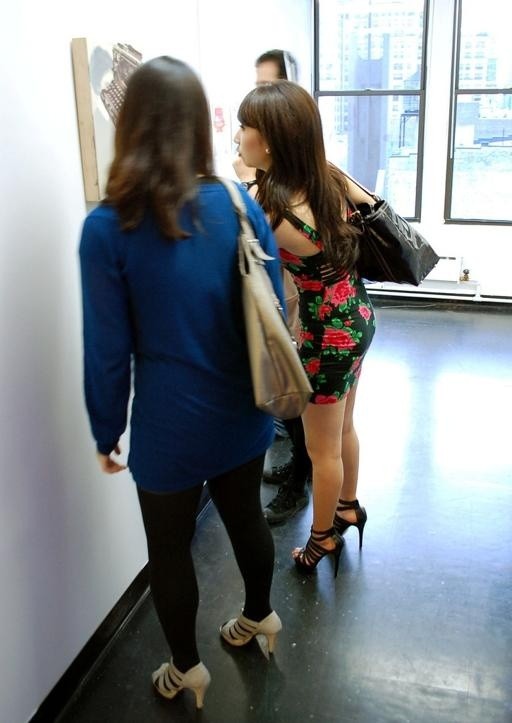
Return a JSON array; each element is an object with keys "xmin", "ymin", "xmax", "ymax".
[{"xmin": 238, "ymin": 179, "xmax": 259, "ymax": 194}]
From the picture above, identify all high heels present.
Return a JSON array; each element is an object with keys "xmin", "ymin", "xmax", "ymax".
[
  {"xmin": 150, "ymin": 657, "xmax": 211, "ymax": 711},
  {"xmin": 217, "ymin": 610, "xmax": 285, "ymax": 655},
  {"xmin": 290, "ymin": 499, "xmax": 368, "ymax": 577}
]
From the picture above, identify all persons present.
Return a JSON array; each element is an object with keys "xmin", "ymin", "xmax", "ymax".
[
  {"xmin": 232, "ymin": 80, "xmax": 380, "ymax": 580},
  {"xmin": 77, "ymin": 52, "xmax": 292, "ymax": 713},
  {"xmin": 231, "ymin": 46, "xmax": 316, "ymax": 528}
]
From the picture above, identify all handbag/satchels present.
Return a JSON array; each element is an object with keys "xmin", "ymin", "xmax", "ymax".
[
  {"xmin": 348, "ymin": 194, "xmax": 441, "ymax": 287},
  {"xmin": 235, "ymin": 234, "xmax": 315, "ymax": 420}
]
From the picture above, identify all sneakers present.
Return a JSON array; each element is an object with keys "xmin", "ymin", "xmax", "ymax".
[{"xmin": 261, "ymin": 462, "xmax": 310, "ymax": 525}]
